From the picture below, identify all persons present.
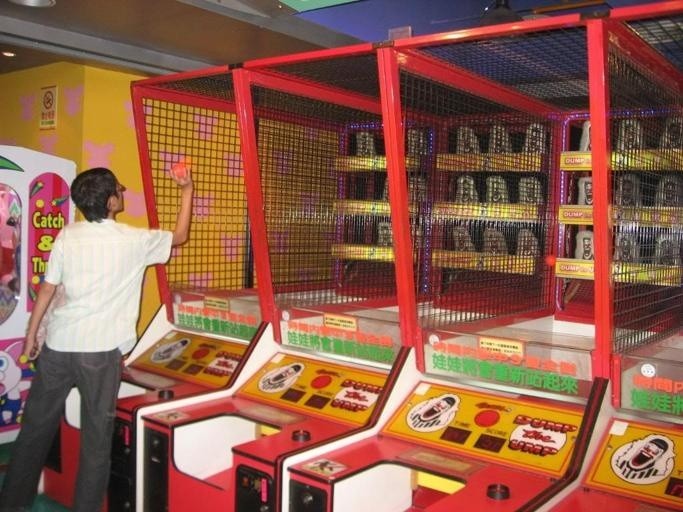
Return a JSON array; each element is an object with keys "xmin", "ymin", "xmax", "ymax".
[{"xmin": 1, "ymin": 161, "xmax": 194, "ymax": 512}]
